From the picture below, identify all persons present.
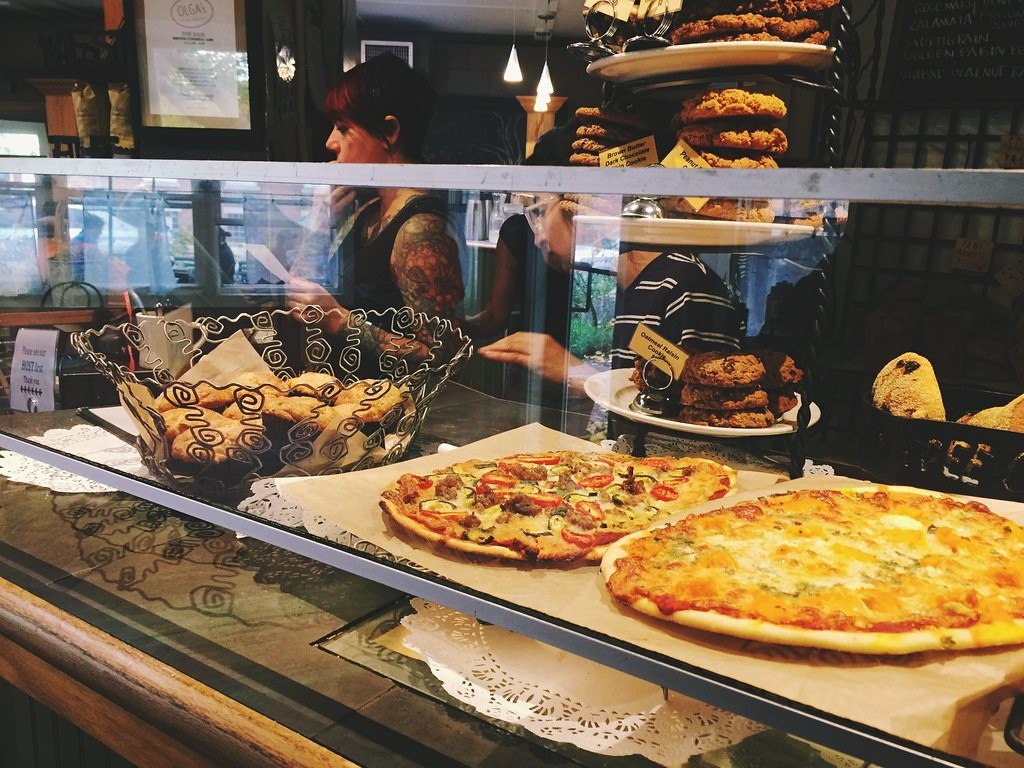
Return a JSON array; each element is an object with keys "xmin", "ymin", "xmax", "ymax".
[
  {"xmin": 257, "ymin": 229, "xmax": 298, "ymax": 286},
  {"xmin": 464, "ymin": 212, "xmax": 569, "ymax": 408},
  {"xmin": 474, "ymin": 126, "xmax": 747, "ymax": 400},
  {"xmin": 69, "ymin": 215, "xmax": 112, "ymax": 284},
  {"xmin": 220, "ymin": 228, "xmax": 236, "ymax": 284},
  {"xmin": 284, "ymin": 52, "xmax": 469, "ymax": 378},
  {"xmin": 125, "ymin": 222, "xmax": 163, "ymax": 282}
]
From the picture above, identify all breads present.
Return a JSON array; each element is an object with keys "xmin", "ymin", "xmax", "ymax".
[
  {"xmin": 148, "ymin": 372, "xmax": 401, "ymax": 464},
  {"xmin": 871, "ymin": 351, "xmax": 1024, "ymax": 465}
]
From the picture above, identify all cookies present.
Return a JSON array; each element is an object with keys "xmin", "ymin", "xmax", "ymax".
[
  {"xmin": 626, "ymin": 351, "xmax": 804, "ymax": 428},
  {"xmin": 564, "ymin": 0, "xmax": 849, "ymax": 228}
]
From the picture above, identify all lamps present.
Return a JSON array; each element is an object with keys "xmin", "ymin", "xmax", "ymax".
[{"xmin": 502, "ymin": 0, "xmax": 555, "ymax": 112}]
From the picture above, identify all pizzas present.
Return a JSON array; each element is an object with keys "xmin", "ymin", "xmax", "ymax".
[
  {"xmin": 378, "ymin": 449, "xmax": 737, "ymax": 565},
  {"xmin": 601, "ymin": 484, "xmax": 1024, "ymax": 654}
]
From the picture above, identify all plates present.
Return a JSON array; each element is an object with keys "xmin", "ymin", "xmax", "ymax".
[
  {"xmin": 572, "ymin": 213, "xmax": 820, "ymax": 248},
  {"xmin": 585, "ymin": 368, "xmax": 822, "ymax": 437},
  {"xmin": 585, "ymin": 40, "xmax": 840, "ymax": 82}
]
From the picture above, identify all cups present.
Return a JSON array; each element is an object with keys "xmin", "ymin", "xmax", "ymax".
[{"xmin": 135, "ymin": 301, "xmax": 209, "ymax": 374}]
